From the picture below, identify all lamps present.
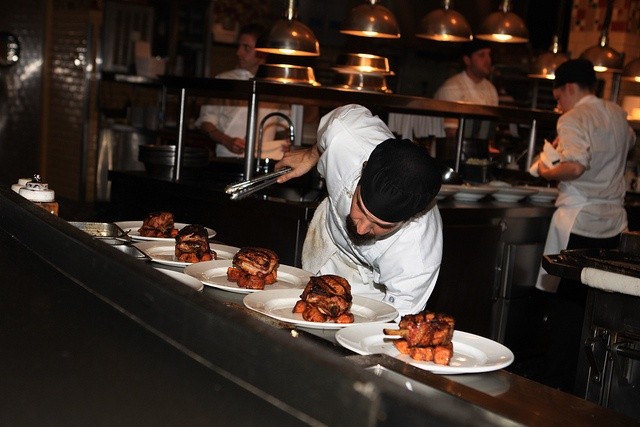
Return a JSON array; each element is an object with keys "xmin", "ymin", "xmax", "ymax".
[
  {"xmin": 578, "ymin": 28, "xmax": 623, "ymax": 74},
  {"xmin": 531, "ymin": 38, "xmax": 568, "ymax": 81},
  {"xmin": 621, "ymin": 57, "xmax": 638, "ymax": 81},
  {"xmin": 256, "ymin": 0, "xmax": 322, "ymax": 56},
  {"xmin": 411, "ymin": 1, "xmax": 474, "ymax": 41},
  {"xmin": 335, "ymin": 1, "xmax": 400, "ymax": 40},
  {"xmin": 477, "ymin": 2, "xmax": 528, "ymax": 44}
]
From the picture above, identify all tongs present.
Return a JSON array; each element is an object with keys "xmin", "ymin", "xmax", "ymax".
[{"xmin": 222, "ymin": 168, "xmax": 300, "ymax": 202}]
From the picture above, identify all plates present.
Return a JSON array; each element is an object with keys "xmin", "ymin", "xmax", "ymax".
[
  {"xmin": 188, "ymin": 259, "xmax": 318, "ymax": 294},
  {"xmin": 493, "ymin": 184, "xmax": 537, "ymax": 207},
  {"xmin": 243, "ymin": 289, "xmax": 400, "ymax": 328},
  {"xmin": 433, "ymin": 186, "xmax": 456, "ymax": 205},
  {"xmin": 522, "ymin": 183, "xmax": 563, "ymax": 204},
  {"xmin": 452, "ymin": 183, "xmax": 497, "ymax": 207},
  {"xmin": 334, "ymin": 322, "xmax": 517, "ymax": 375},
  {"xmin": 145, "ymin": 244, "xmax": 239, "ymax": 269},
  {"xmin": 115, "ymin": 217, "xmax": 218, "ymax": 243}
]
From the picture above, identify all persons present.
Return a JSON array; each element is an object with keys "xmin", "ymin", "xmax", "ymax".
[
  {"xmin": 273, "ymin": 104, "xmax": 444, "ymax": 317},
  {"xmin": 195, "ymin": 23, "xmax": 292, "ymax": 251},
  {"xmin": 433, "ymin": 41, "xmax": 500, "ymax": 179},
  {"xmin": 522, "ymin": 58, "xmax": 636, "ymax": 384}
]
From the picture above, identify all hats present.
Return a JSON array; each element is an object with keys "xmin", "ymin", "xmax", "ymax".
[
  {"xmin": 552, "ymin": 59, "xmax": 596, "ymax": 90},
  {"xmin": 361, "ymin": 138, "xmax": 442, "ymax": 224}
]
